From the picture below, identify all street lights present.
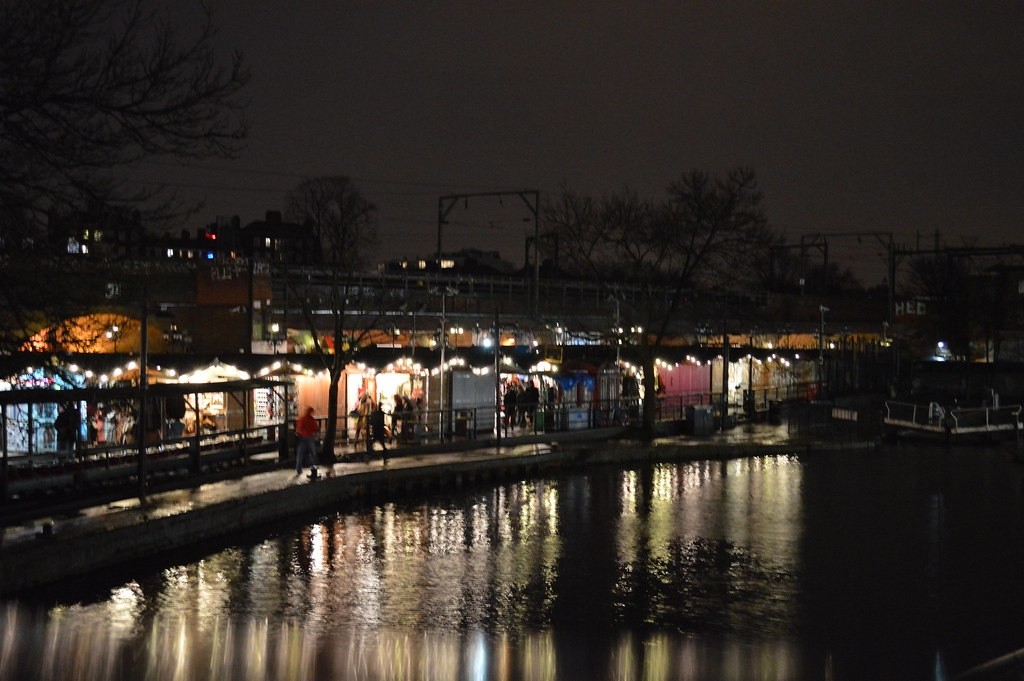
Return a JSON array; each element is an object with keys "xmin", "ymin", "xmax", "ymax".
[
  {"xmin": 817, "ymin": 303, "xmax": 830, "ymax": 393},
  {"xmin": 106, "ymin": 280, "xmax": 146, "ymax": 490},
  {"xmin": 436, "ymin": 285, "xmax": 453, "ymax": 443}
]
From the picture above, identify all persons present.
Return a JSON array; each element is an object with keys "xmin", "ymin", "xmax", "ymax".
[
  {"xmin": 91, "ymin": 410, "xmax": 105, "ymax": 448},
  {"xmin": 388, "ymin": 394, "xmax": 425, "ymax": 444},
  {"xmin": 294, "ymin": 406, "xmax": 322, "ymax": 478},
  {"xmin": 369, "ymin": 402, "xmax": 387, "ymax": 451},
  {"xmin": 53, "ymin": 402, "xmax": 81, "ymax": 453},
  {"xmin": 351, "ymin": 392, "xmax": 377, "ymax": 449},
  {"xmin": 503, "ymin": 380, "xmax": 555, "ymax": 437},
  {"xmin": 621, "ymin": 372, "xmax": 640, "ymax": 418}
]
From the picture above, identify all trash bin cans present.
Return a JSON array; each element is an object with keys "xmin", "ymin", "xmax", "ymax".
[{"xmin": 687, "ymin": 404, "xmax": 716, "ymax": 438}]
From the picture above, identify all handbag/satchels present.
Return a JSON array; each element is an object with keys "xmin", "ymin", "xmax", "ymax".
[{"xmin": 287, "ymin": 432, "xmax": 301, "ymax": 446}]
